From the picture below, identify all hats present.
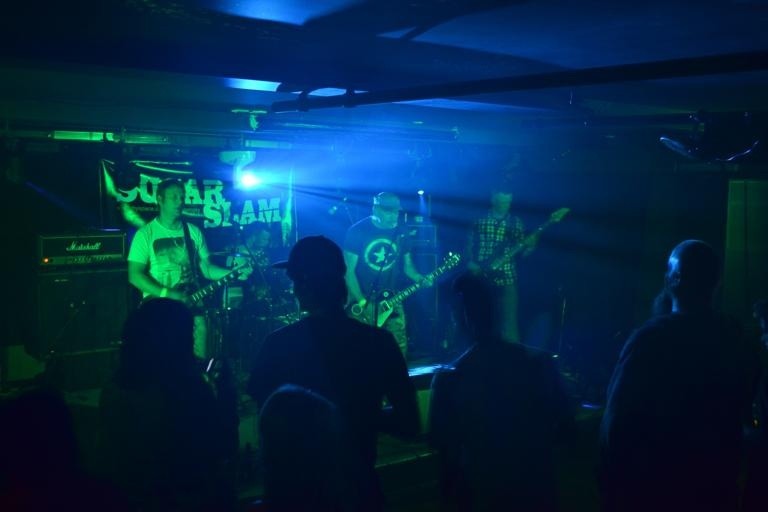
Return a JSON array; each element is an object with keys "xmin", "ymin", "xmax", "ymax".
[
  {"xmin": 267, "ymin": 233, "xmax": 350, "ymax": 277},
  {"xmin": 371, "ymin": 191, "xmax": 407, "ymax": 214},
  {"xmin": 663, "ymin": 236, "xmax": 725, "ymax": 286}
]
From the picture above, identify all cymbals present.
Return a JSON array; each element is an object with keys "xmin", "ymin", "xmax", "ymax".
[{"xmin": 272, "ymin": 261, "xmax": 292, "ymax": 270}]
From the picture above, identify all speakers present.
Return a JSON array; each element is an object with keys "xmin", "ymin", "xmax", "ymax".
[
  {"xmin": 397, "ymin": 252, "xmax": 438, "ymax": 320},
  {"xmin": 24, "ymin": 266, "xmax": 130, "ymax": 360}
]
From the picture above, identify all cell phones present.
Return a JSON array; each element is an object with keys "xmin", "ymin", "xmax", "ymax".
[{"xmin": 202, "ymin": 359, "xmax": 216, "ymax": 374}]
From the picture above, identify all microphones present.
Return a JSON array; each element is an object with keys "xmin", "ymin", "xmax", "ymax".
[{"xmin": 228, "ymin": 216, "xmax": 243, "ymax": 231}]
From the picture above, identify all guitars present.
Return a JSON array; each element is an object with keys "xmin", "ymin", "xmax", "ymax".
[
  {"xmin": 136, "ymin": 251, "xmax": 268, "ymax": 317},
  {"xmin": 346, "ymin": 249, "xmax": 462, "ymax": 330},
  {"xmin": 447, "ymin": 208, "xmax": 568, "ymax": 311}
]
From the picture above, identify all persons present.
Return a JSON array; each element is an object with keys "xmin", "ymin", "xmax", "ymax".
[
  {"xmin": 341, "ymin": 188, "xmax": 434, "ymax": 361},
  {"xmin": 2, "ymin": 232, "xmax": 765, "ymax": 511},
  {"xmin": 462, "ymin": 182, "xmax": 542, "ymax": 342},
  {"xmin": 122, "ymin": 176, "xmax": 252, "ymax": 361}
]
